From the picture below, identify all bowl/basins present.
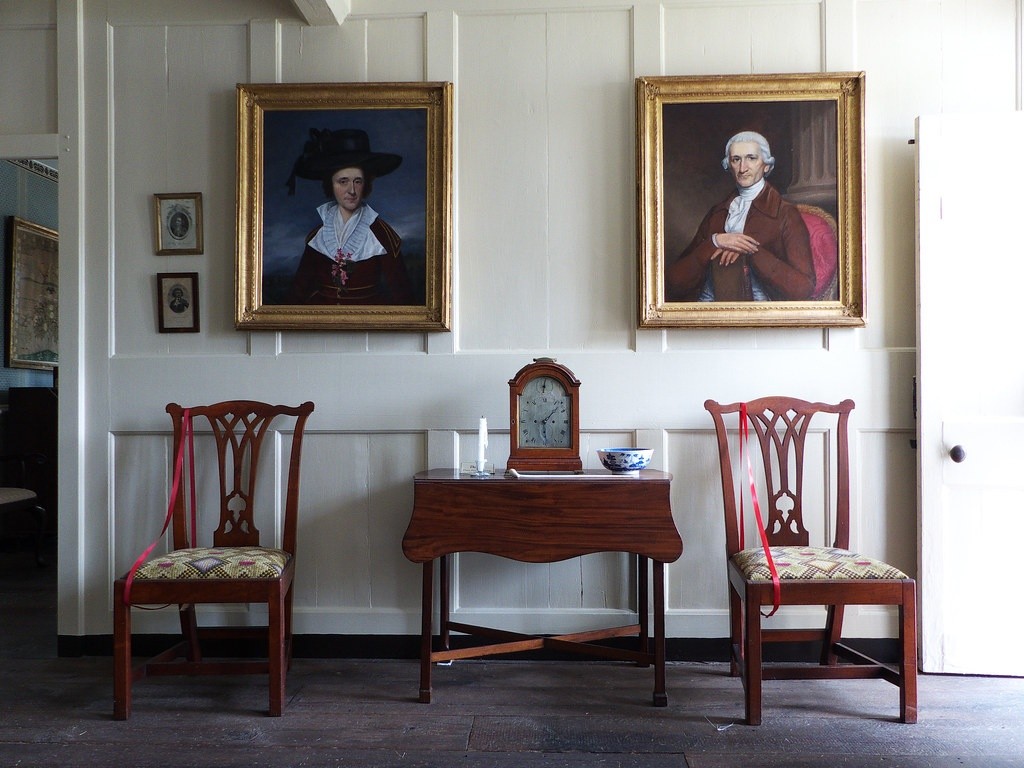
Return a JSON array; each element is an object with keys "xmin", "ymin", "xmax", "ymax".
[{"xmin": 596, "ymin": 448, "xmax": 654, "ymax": 475}]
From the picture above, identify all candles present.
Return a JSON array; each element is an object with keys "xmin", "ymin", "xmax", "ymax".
[
  {"xmin": 479, "ymin": 416, "xmax": 488, "ymax": 471},
  {"xmin": 454, "ymin": 432, "xmax": 460, "ymax": 468}
]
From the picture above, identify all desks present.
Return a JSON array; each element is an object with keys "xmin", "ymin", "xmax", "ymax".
[{"xmin": 401, "ymin": 468, "xmax": 683, "ymax": 706}]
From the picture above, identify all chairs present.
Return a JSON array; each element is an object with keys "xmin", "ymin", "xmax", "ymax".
[
  {"xmin": 113, "ymin": 401, "xmax": 315, "ymax": 721},
  {"xmin": 705, "ymin": 397, "xmax": 917, "ymax": 726}
]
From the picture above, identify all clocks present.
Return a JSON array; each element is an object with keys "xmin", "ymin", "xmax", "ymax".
[{"xmin": 508, "ymin": 358, "xmax": 585, "ymax": 475}]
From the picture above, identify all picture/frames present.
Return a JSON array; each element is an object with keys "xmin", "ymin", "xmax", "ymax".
[
  {"xmin": 157, "ymin": 272, "xmax": 200, "ymax": 334},
  {"xmin": 635, "ymin": 71, "xmax": 868, "ymax": 329},
  {"xmin": 235, "ymin": 82, "xmax": 453, "ymax": 335},
  {"xmin": 4, "ymin": 215, "xmax": 59, "ymax": 372},
  {"xmin": 155, "ymin": 193, "xmax": 204, "ymax": 256}
]
